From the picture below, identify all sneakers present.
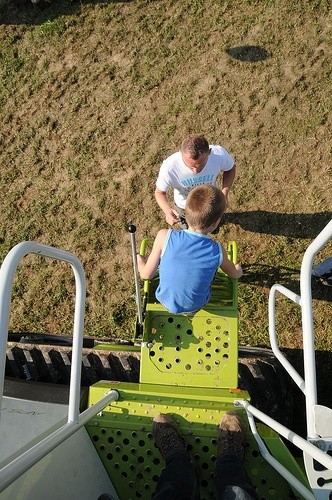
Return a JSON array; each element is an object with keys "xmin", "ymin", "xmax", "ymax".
[
  {"xmin": 218, "ymin": 412, "xmax": 246, "ymax": 463},
  {"xmin": 150, "ymin": 414, "xmax": 188, "ymax": 462}
]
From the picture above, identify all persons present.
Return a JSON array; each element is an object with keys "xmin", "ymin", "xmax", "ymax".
[
  {"xmin": 152, "ymin": 412, "xmax": 257, "ymax": 500},
  {"xmin": 154, "ymin": 136, "xmax": 235, "ymax": 234},
  {"xmin": 137, "ymin": 184, "xmax": 243, "ymax": 315},
  {"xmin": 311, "ymin": 257, "xmax": 332, "ymax": 288}
]
